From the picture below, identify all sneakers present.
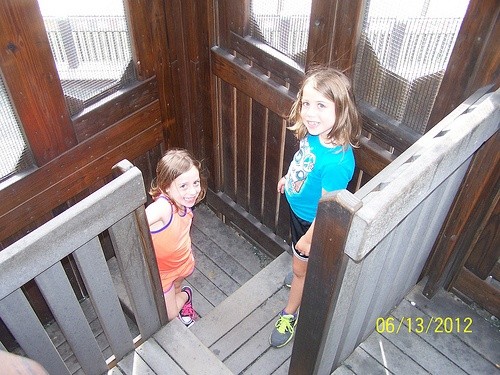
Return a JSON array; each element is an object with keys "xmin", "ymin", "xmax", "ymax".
[
  {"xmin": 284, "ymin": 272, "xmax": 294, "ymax": 287},
  {"xmin": 178, "ymin": 286, "xmax": 195, "ymax": 326},
  {"xmin": 270, "ymin": 304, "xmax": 300, "ymax": 348}
]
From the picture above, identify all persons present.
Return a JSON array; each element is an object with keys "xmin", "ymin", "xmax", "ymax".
[
  {"xmin": 143, "ymin": 145, "xmax": 211, "ymax": 326},
  {"xmin": 269, "ymin": 43, "xmax": 372, "ymax": 347}
]
